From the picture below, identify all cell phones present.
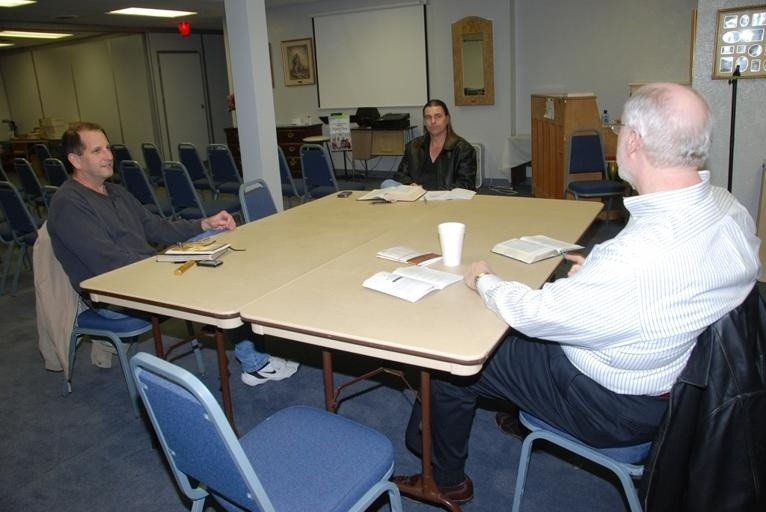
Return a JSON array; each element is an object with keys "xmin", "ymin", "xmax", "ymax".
[{"xmin": 197, "ymin": 258, "xmax": 223, "ymax": 267}]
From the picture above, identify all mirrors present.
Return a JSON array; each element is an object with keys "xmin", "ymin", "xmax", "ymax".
[{"xmin": 451, "ymin": 16, "xmax": 495, "ymax": 106}]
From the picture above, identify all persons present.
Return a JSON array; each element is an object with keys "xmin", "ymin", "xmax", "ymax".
[
  {"xmin": 392, "ymin": 99, "xmax": 477, "ymax": 195},
  {"xmin": 47, "ymin": 123, "xmax": 301, "ymax": 388},
  {"xmin": 389, "ymin": 82, "xmax": 761, "ymax": 503}
]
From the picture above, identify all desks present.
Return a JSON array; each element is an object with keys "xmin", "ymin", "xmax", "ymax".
[
  {"xmin": 349, "ymin": 125, "xmax": 417, "ymax": 184},
  {"xmin": 240, "ymin": 193, "xmax": 605, "ymax": 512},
  {"xmin": 80, "ymin": 189, "xmax": 446, "ymax": 438}
]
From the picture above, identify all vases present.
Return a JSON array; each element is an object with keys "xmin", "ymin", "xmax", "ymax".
[{"xmin": 231, "ymin": 110, "xmax": 238, "ymax": 128}]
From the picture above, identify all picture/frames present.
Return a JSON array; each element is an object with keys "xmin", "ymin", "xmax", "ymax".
[
  {"xmin": 279, "ymin": 37, "xmax": 315, "ymax": 87},
  {"xmin": 711, "ymin": 4, "xmax": 765, "ymax": 78}
]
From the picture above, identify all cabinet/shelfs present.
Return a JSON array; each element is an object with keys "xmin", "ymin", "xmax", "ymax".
[{"xmin": 223, "ymin": 123, "xmax": 324, "ymax": 178}]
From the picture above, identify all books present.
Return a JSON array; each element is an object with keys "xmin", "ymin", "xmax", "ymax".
[
  {"xmin": 362, "ymin": 244, "xmax": 464, "ymax": 304},
  {"xmin": 357, "ymin": 186, "xmax": 427, "ymax": 203},
  {"xmin": 489, "ymin": 234, "xmax": 587, "ymax": 265},
  {"xmin": 154, "ymin": 241, "xmax": 231, "ymax": 263}
]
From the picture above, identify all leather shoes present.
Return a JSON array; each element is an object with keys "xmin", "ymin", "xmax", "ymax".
[
  {"xmin": 495, "ymin": 409, "xmax": 546, "ymax": 453},
  {"xmin": 391, "ymin": 474, "xmax": 474, "ymax": 504}
]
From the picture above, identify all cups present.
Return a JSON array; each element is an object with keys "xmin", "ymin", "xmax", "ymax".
[{"xmin": 437, "ymin": 222, "xmax": 465, "ymax": 267}]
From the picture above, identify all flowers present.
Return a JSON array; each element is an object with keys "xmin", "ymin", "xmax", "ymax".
[{"xmin": 226, "ymin": 94, "xmax": 235, "ymax": 112}]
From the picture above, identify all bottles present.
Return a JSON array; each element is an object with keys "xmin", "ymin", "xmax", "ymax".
[{"xmin": 602, "ymin": 110, "xmax": 609, "ymax": 127}]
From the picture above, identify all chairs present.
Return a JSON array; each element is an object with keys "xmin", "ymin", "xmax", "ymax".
[
  {"xmin": 0, "ymin": 143, "xmax": 364, "ymax": 297},
  {"xmin": 129, "ymin": 349, "xmax": 405, "ymax": 511},
  {"xmin": 66, "ymin": 293, "xmax": 209, "ymax": 418},
  {"xmin": 511, "ymin": 411, "xmax": 652, "ymax": 512},
  {"xmin": 563, "ymin": 126, "xmax": 632, "ymax": 224},
  {"xmin": 467, "ymin": 142, "xmax": 483, "ymax": 188}
]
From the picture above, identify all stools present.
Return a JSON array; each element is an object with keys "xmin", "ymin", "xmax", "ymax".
[{"xmin": 303, "ymin": 136, "xmax": 336, "ymax": 177}]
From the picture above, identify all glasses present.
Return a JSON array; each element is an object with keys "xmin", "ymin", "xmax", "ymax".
[{"xmin": 607, "ymin": 118, "xmax": 630, "ymax": 136}]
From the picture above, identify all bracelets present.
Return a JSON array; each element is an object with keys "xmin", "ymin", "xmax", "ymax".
[{"xmin": 473, "ymin": 270, "xmax": 493, "ymax": 294}]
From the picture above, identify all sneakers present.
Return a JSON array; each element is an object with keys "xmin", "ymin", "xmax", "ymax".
[{"xmin": 241, "ymin": 356, "xmax": 301, "ymax": 387}]
